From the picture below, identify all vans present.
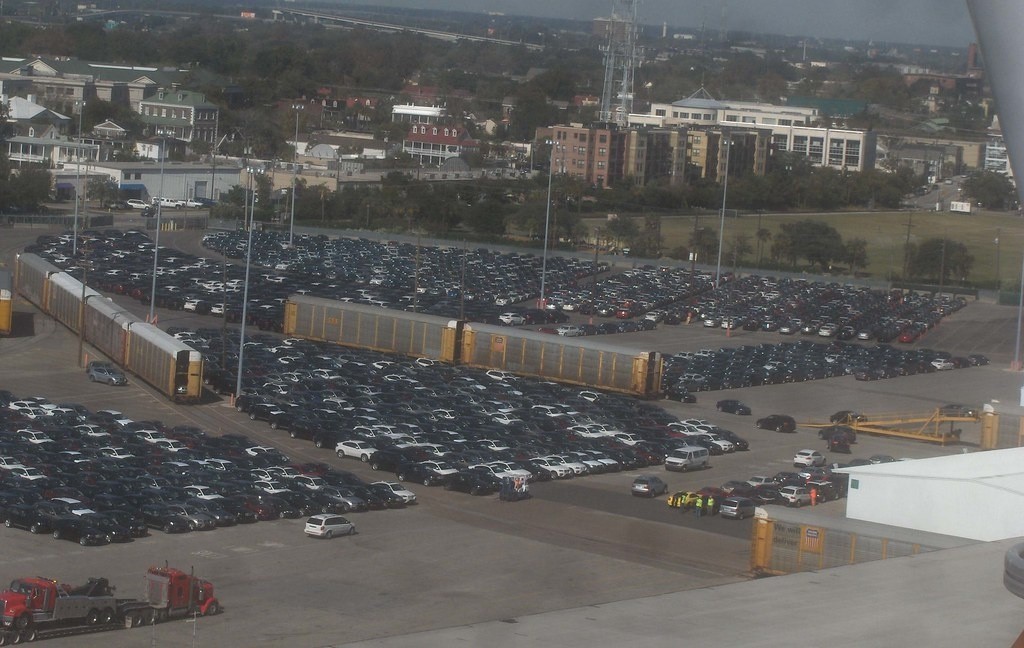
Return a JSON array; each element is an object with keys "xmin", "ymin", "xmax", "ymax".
[{"xmin": 665, "ymin": 446, "xmax": 710, "ymax": 473}]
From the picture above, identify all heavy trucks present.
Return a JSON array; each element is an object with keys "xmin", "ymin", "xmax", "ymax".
[{"xmin": 0, "ymin": 561, "xmax": 220, "ymax": 648}]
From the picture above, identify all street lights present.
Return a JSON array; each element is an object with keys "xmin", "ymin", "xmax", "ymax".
[
  {"xmin": 288, "ymin": 104, "xmax": 305, "ymax": 246},
  {"xmin": 538, "ymin": 138, "xmax": 562, "ymax": 308},
  {"xmin": 71, "ymin": 100, "xmax": 87, "ymax": 256},
  {"xmin": 235, "ymin": 167, "xmax": 266, "ymax": 398},
  {"xmin": 148, "ymin": 128, "xmax": 179, "ymax": 321},
  {"xmin": 714, "ymin": 139, "xmax": 735, "ymax": 289}
]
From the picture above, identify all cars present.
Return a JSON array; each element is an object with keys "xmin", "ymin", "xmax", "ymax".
[
  {"xmin": 303, "ymin": 513, "xmax": 357, "ymax": 541},
  {"xmin": 1, "ymin": 196, "xmax": 991, "ymax": 546},
  {"xmin": 631, "ymin": 474, "xmax": 669, "ymax": 499}
]
